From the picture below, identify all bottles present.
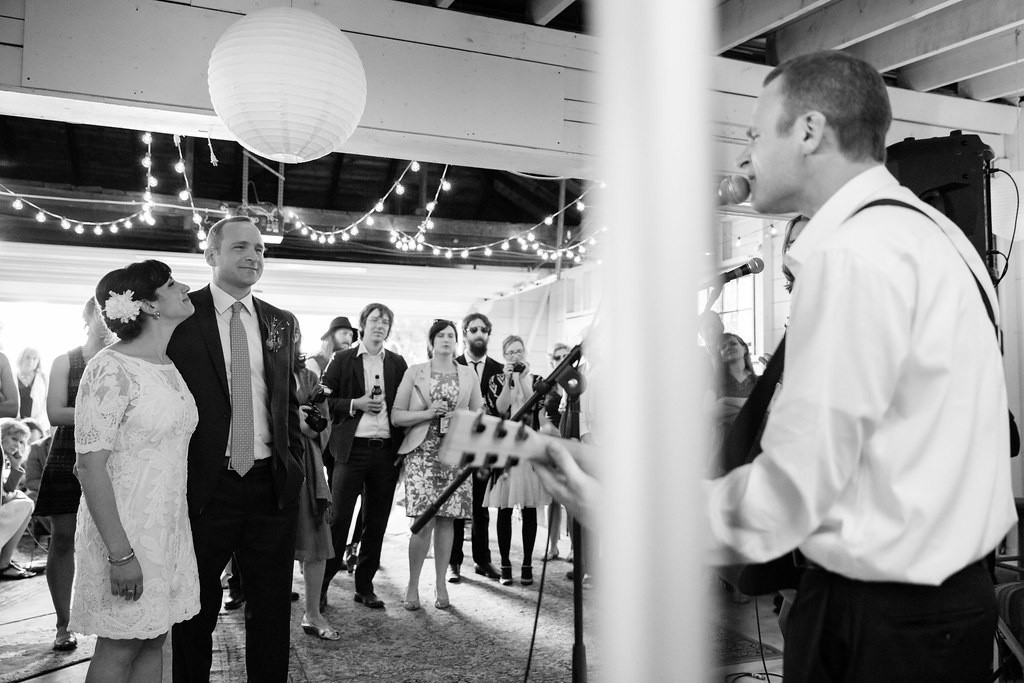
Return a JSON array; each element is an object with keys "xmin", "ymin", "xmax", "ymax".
[
  {"xmin": 438, "ymin": 397, "xmax": 449, "ymax": 437},
  {"xmin": 370, "ymin": 374, "xmax": 382, "ymax": 414}
]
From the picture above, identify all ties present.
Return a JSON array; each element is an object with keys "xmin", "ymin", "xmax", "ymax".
[
  {"xmin": 229, "ymin": 301, "xmax": 255, "ymax": 478},
  {"xmin": 474, "ymin": 362, "xmax": 479, "ymax": 376}
]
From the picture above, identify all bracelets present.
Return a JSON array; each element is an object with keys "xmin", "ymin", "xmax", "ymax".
[{"xmin": 106, "ymin": 548, "xmax": 135, "ymax": 566}]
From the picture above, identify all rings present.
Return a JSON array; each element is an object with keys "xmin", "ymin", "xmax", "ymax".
[{"xmin": 126, "ymin": 590, "xmax": 135, "ymax": 595}]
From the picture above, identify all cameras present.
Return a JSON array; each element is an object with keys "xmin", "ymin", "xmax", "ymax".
[
  {"xmin": 509, "ymin": 362, "xmax": 525, "ymax": 373},
  {"xmin": 302, "ymin": 383, "xmax": 333, "ymax": 432}
]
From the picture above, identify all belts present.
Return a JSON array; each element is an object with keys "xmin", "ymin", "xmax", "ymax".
[
  {"xmin": 223, "ymin": 457, "xmax": 273, "ymax": 471},
  {"xmin": 353, "ymin": 437, "xmax": 391, "ymax": 448}
]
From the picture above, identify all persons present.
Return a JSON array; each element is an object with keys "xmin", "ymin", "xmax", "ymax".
[
  {"xmin": 531, "ymin": 48, "xmax": 1019, "ymax": 683},
  {"xmin": 166, "ymin": 215, "xmax": 306, "ymax": 683},
  {"xmin": 68, "ymin": 260, "xmax": 202, "ymax": 683},
  {"xmin": 0, "ymin": 216, "xmax": 811, "ymax": 651}
]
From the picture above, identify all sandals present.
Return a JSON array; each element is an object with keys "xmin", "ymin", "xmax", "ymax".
[{"xmin": 0, "ymin": 560, "xmax": 36, "ymax": 580}]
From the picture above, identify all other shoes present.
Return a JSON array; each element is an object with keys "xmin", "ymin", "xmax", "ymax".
[
  {"xmin": 566, "ymin": 549, "xmax": 574, "ymax": 563},
  {"xmin": 340, "ymin": 560, "xmax": 348, "ymax": 570},
  {"xmin": 225, "ymin": 599, "xmax": 245, "ymax": 609},
  {"xmin": 447, "ymin": 561, "xmax": 460, "ymax": 583},
  {"xmin": 291, "ymin": 592, "xmax": 299, "ymax": 601},
  {"xmin": 566, "ymin": 571, "xmax": 574, "ymax": 579},
  {"xmin": 54, "ymin": 630, "xmax": 77, "ymax": 648},
  {"xmin": 474, "ymin": 562, "xmax": 502, "ymax": 579},
  {"xmin": 546, "ymin": 548, "xmax": 559, "ymax": 561},
  {"xmin": 320, "ymin": 593, "xmax": 326, "ymax": 612},
  {"xmin": 354, "ymin": 591, "xmax": 385, "ymax": 607}
]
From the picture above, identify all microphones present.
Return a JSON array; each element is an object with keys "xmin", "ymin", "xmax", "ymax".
[
  {"xmin": 721, "ymin": 257, "xmax": 764, "ymax": 282},
  {"xmin": 716, "ymin": 175, "xmax": 750, "ymax": 206}
]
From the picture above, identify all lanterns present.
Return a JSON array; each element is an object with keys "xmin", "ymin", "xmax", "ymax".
[{"xmin": 208, "ymin": 8, "xmax": 366, "ymax": 163}]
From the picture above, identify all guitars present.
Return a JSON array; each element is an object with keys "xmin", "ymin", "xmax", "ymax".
[{"xmin": 435, "ymin": 393, "xmax": 809, "ymax": 596}]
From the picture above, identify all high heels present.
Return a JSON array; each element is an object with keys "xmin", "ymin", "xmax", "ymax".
[
  {"xmin": 404, "ymin": 585, "xmax": 420, "ymax": 610},
  {"xmin": 521, "ymin": 560, "xmax": 532, "ymax": 585},
  {"xmin": 435, "ymin": 587, "xmax": 449, "ymax": 608},
  {"xmin": 499, "ymin": 560, "xmax": 512, "ymax": 586},
  {"xmin": 300, "ymin": 612, "xmax": 341, "ymax": 640}
]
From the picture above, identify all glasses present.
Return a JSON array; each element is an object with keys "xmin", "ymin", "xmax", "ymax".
[
  {"xmin": 554, "ymin": 354, "xmax": 568, "ymax": 359},
  {"xmin": 504, "ymin": 348, "xmax": 524, "ymax": 356},
  {"xmin": 465, "ymin": 327, "xmax": 490, "ymax": 333},
  {"xmin": 369, "ymin": 319, "xmax": 392, "ymax": 327}
]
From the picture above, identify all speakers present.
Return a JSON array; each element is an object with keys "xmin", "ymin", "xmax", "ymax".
[{"xmin": 885, "ymin": 129, "xmax": 994, "ymax": 266}]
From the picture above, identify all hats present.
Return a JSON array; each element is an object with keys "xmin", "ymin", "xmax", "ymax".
[{"xmin": 321, "ymin": 316, "xmax": 358, "ymax": 344}]
